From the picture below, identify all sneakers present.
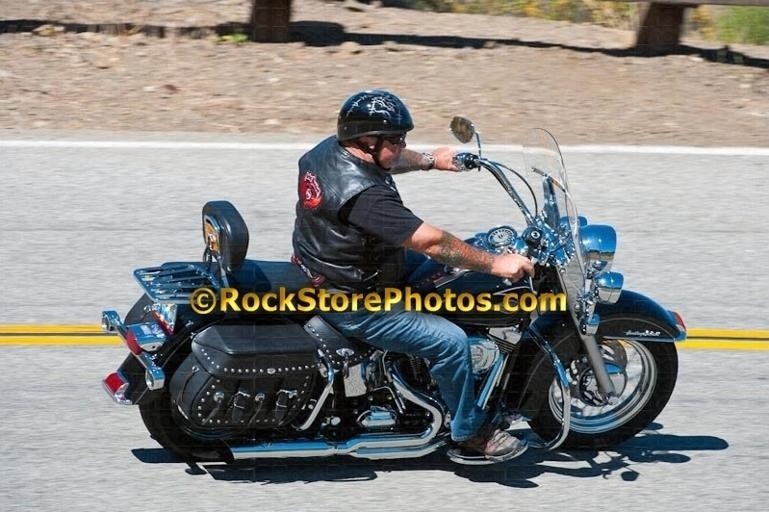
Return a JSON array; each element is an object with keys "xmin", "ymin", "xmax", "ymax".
[{"xmin": 456, "ymin": 419, "xmax": 530, "ymax": 461}]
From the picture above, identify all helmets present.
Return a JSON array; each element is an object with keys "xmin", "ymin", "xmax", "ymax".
[{"xmin": 336, "ymin": 89, "xmax": 414, "ymax": 145}]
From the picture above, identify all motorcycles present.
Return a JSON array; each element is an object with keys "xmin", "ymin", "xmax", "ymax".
[{"xmin": 99, "ymin": 114, "xmax": 690, "ymax": 480}]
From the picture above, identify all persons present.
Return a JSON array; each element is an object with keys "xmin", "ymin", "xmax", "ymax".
[{"xmin": 292, "ymin": 90, "xmax": 536, "ymax": 464}]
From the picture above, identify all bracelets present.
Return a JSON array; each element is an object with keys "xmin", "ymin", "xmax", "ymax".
[{"xmin": 421, "ymin": 150, "xmax": 436, "ymax": 171}]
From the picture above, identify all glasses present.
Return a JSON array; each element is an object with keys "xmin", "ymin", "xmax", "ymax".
[{"xmin": 368, "ymin": 134, "xmax": 406, "ymax": 144}]
